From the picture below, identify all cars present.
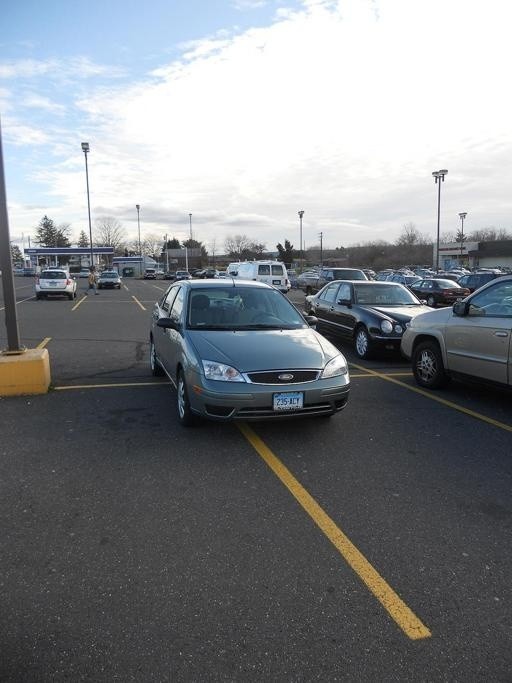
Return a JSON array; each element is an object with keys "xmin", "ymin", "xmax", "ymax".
[
  {"xmin": 97, "ymin": 271, "xmax": 121, "ymax": 289},
  {"xmin": 80, "ymin": 269, "xmax": 91, "ymax": 278},
  {"xmin": 144, "ymin": 268, "xmax": 227, "ymax": 280},
  {"xmin": 149, "ymin": 278, "xmax": 351, "ymax": 427},
  {"xmin": 288, "ymin": 267, "xmax": 512, "ymax": 292},
  {"xmin": 303, "ymin": 278, "xmax": 435, "ymax": 359},
  {"xmin": 407, "ymin": 279, "xmax": 471, "ymax": 306},
  {"xmin": 399, "ymin": 273, "xmax": 512, "ymax": 388},
  {"xmin": 24, "ymin": 267, "xmax": 35, "ymax": 277}
]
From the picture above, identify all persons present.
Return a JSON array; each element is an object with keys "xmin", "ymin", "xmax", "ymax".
[
  {"xmin": 239, "ymin": 294, "xmax": 271, "ymax": 325},
  {"xmin": 84, "ymin": 270, "xmax": 100, "ymax": 295}
]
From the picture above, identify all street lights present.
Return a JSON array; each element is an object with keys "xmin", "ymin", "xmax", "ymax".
[
  {"xmin": 432, "ymin": 169, "xmax": 448, "ymax": 270},
  {"xmin": 458, "ymin": 212, "xmax": 467, "ymax": 267},
  {"xmin": 81, "ymin": 141, "xmax": 94, "ymax": 267},
  {"xmin": 136, "ymin": 204, "xmax": 142, "ymax": 258},
  {"xmin": 297, "ymin": 210, "xmax": 305, "ymax": 270}
]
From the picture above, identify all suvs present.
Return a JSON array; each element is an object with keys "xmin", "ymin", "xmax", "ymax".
[{"xmin": 35, "ymin": 268, "xmax": 77, "ymax": 300}]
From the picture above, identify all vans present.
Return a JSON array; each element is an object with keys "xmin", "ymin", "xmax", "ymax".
[{"xmin": 226, "ymin": 261, "xmax": 291, "ymax": 293}]
